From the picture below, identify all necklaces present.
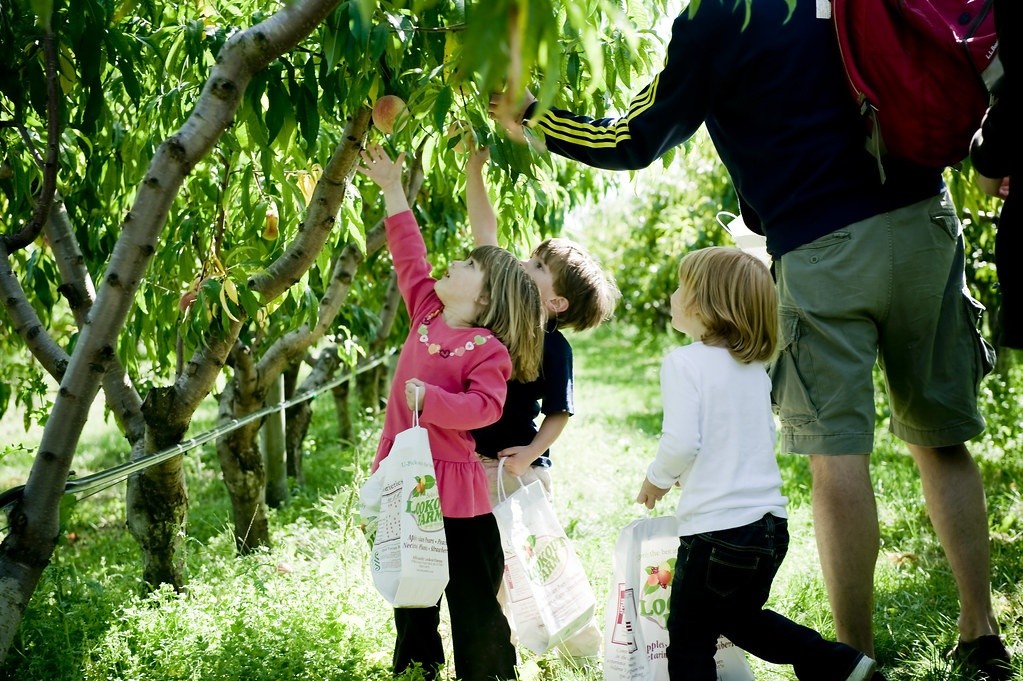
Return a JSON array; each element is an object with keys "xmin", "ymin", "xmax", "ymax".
[{"xmin": 418, "ymin": 308, "xmax": 494, "ymax": 358}]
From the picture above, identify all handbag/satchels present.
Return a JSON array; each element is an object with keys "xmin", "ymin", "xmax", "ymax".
[
  {"xmin": 602, "ymin": 502, "xmax": 754, "ymax": 681},
  {"xmin": 493, "ymin": 455, "xmax": 595, "ymax": 655},
  {"xmin": 357, "ymin": 386, "xmax": 450, "ymax": 608}
]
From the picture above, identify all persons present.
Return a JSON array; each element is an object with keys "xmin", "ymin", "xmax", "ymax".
[
  {"xmin": 356, "ymin": 144, "xmax": 544, "ymax": 681},
  {"xmin": 635, "ymin": 247, "xmax": 877, "ymax": 681},
  {"xmin": 465, "ymin": 143, "xmax": 618, "ymax": 674},
  {"xmin": 486, "ymin": 0, "xmax": 1023, "ymax": 681}
]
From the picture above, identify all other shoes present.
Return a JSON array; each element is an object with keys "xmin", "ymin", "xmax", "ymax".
[
  {"xmin": 946, "ymin": 634, "xmax": 1013, "ymax": 679},
  {"xmin": 845, "ymin": 653, "xmax": 877, "ymax": 681}
]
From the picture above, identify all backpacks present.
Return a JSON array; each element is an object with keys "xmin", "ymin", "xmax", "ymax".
[{"xmin": 832, "ymin": 0, "xmax": 991, "ymax": 168}]
